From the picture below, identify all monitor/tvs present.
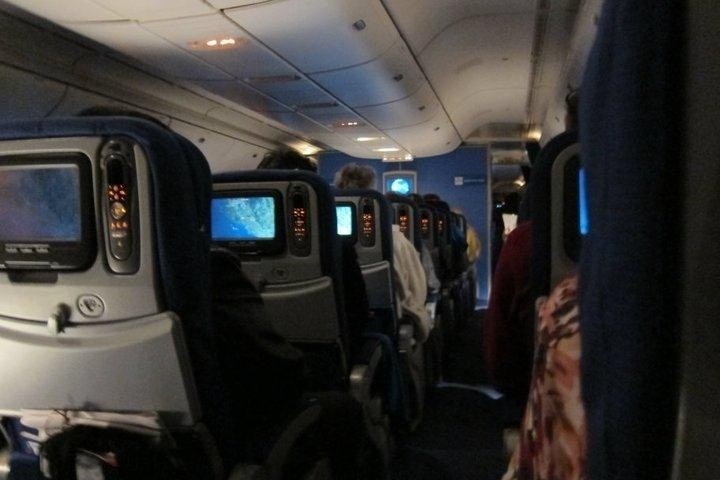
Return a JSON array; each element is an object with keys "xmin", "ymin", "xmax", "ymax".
[
  {"xmin": 331, "ymin": 201, "xmax": 358, "ymax": 244},
  {"xmin": 382, "ymin": 171, "xmax": 417, "ymax": 195},
  {"xmin": 0, "ymin": 150, "xmax": 97, "ymax": 272},
  {"xmin": 560, "ymin": 155, "xmax": 592, "ymax": 265},
  {"xmin": 207, "ymin": 188, "xmax": 285, "ymax": 260}
]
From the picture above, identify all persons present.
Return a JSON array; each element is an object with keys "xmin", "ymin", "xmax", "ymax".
[{"xmin": 77, "ymin": 91, "xmax": 592, "ymax": 480}]
